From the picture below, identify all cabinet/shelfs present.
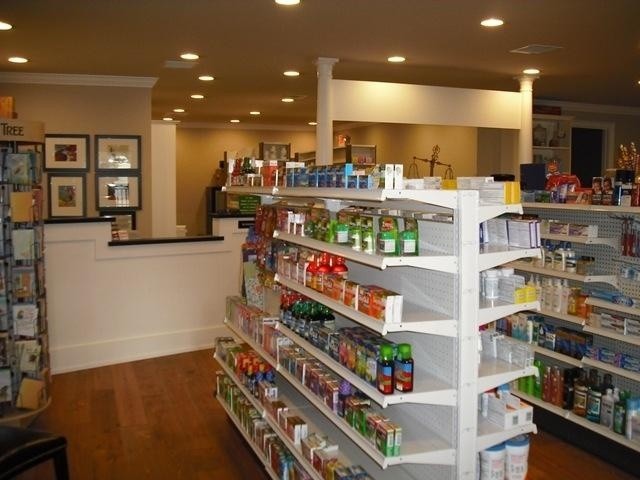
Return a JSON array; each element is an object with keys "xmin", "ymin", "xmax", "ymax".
[{"xmin": 532, "ymin": 146, "xmax": 570, "ymax": 174}]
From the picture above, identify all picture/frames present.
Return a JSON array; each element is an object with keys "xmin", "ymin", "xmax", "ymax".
[{"xmin": 42, "ymin": 133, "xmax": 142, "ymax": 229}]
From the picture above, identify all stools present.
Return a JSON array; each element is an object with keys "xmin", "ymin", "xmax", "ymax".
[{"xmin": 1, "ymin": 426, "xmax": 69, "ymax": 480}]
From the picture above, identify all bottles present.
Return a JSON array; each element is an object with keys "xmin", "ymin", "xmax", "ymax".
[
  {"xmin": 376, "ymin": 345, "xmax": 394, "ymax": 396},
  {"xmin": 236, "ymin": 350, "xmax": 276, "ymax": 398},
  {"xmin": 478, "ymin": 266, "xmax": 515, "ymax": 299},
  {"xmin": 394, "ymin": 343, "xmax": 416, "ymax": 391},
  {"xmin": 280, "ymin": 292, "xmax": 335, "ymax": 342},
  {"xmin": 305, "ymin": 252, "xmax": 347, "ymax": 293},
  {"xmin": 549, "ymin": 130, "xmax": 560, "ymax": 147},
  {"xmin": 231, "ymin": 156, "xmax": 257, "ymax": 187}
]
